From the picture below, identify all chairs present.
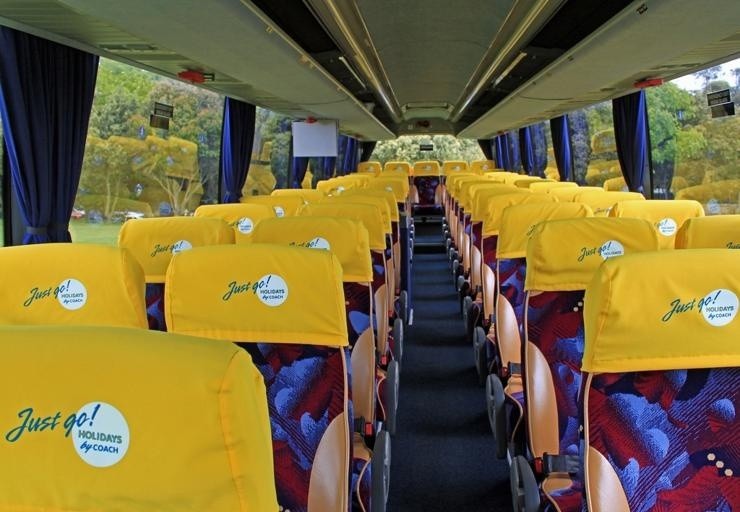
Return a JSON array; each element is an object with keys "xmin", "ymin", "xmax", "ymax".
[
  {"xmin": 442, "ymin": 171, "xmax": 740, "ymax": 512},
  {"xmin": 356, "ymin": 163, "xmax": 497, "ymax": 205},
  {"xmin": 1, "ymin": 170, "xmax": 416, "ymax": 510}
]
